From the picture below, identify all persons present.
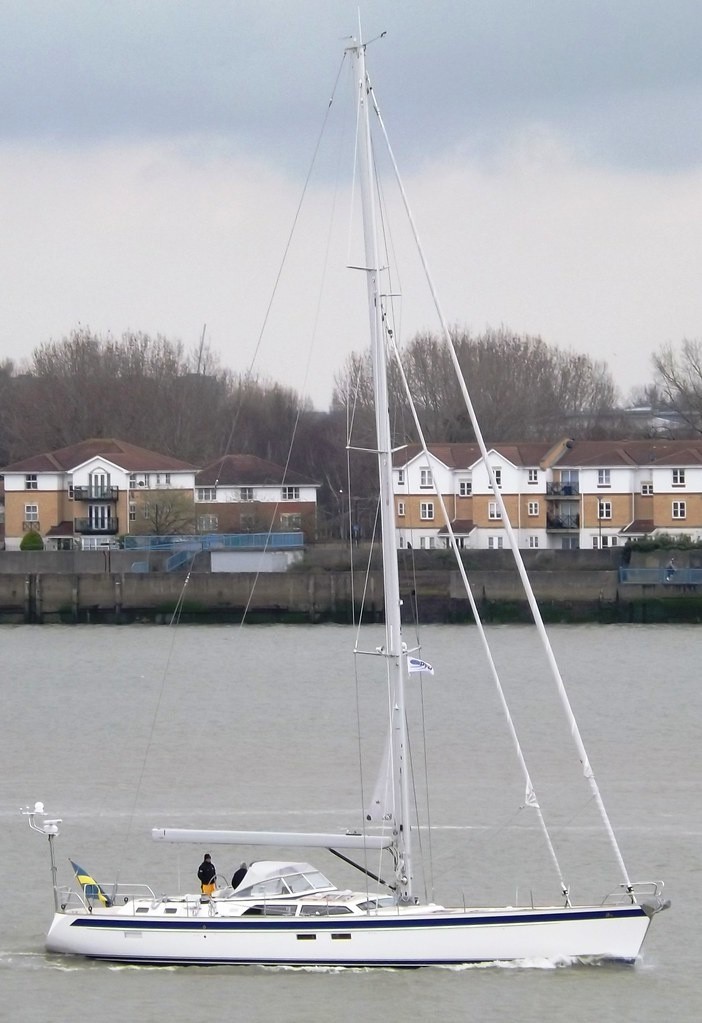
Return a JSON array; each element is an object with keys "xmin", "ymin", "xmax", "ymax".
[
  {"xmin": 231, "ymin": 862, "xmax": 253, "ymax": 891},
  {"xmin": 197, "ymin": 854, "xmax": 217, "ymax": 898},
  {"xmin": 665, "ymin": 557, "xmax": 677, "ymax": 581}
]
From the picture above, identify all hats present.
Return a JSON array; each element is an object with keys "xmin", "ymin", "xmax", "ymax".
[
  {"xmin": 240, "ymin": 862, "xmax": 247, "ymax": 869},
  {"xmin": 204, "ymin": 854, "xmax": 212, "ymax": 861}
]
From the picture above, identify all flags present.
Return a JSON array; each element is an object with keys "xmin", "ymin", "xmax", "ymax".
[
  {"xmin": 69, "ymin": 858, "xmax": 113, "ymax": 907},
  {"xmin": 407, "ymin": 656, "xmax": 435, "ymax": 679}
]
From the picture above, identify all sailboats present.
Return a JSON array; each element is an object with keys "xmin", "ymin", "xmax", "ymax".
[{"xmin": 26, "ymin": 11, "xmax": 672, "ymax": 973}]
